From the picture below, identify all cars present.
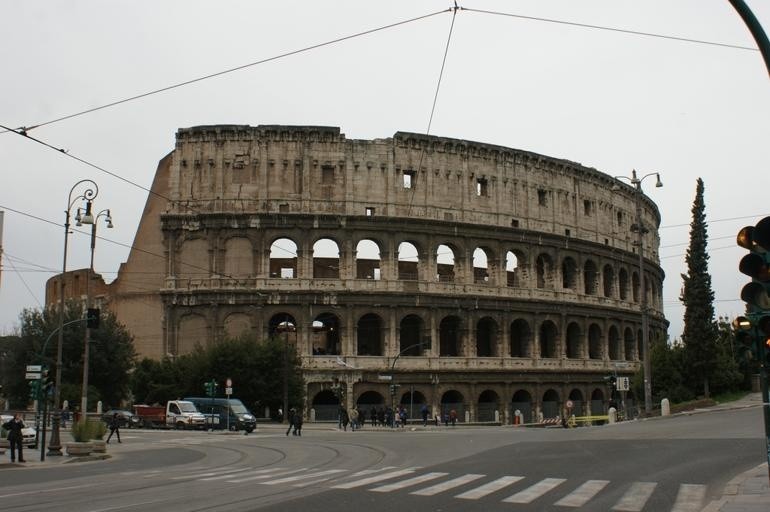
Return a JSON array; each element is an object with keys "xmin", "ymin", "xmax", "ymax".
[
  {"xmin": 0, "ymin": 415, "xmax": 37, "ymax": 448},
  {"xmin": 100, "ymin": 409, "xmax": 145, "ymax": 429}
]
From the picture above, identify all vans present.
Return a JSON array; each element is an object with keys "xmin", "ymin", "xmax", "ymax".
[{"xmin": 183, "ymin": 397, "xmax": 256, "ymax": 433}]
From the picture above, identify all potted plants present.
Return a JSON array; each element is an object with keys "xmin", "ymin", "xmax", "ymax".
[
  {"xmin": 66, "ymin": 420, "xmax": 95, "ymax": 456},
  {"xmin": 89, "ymin": 421, "xmax": 107, "ymax": 453}
]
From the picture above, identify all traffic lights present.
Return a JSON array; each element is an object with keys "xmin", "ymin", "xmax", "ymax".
[
  {"xmin": 204, "ymin": 383, "xmax": 211, "ymax": 396},
  {"xmin": 41, "ymin": 369, "xmax": 54, "ymax": 399},
  {"xmin": 732, "ymin": 216, "xmax": 770, "ymax": 373},
  {"xmin": 391, "ymin": 384, "xmax": 400, "ymax": 398}
]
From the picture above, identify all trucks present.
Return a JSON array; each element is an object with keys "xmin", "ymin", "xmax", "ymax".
[{"xmin": 132, "ymin": 400, "xmax": 209, "ymax": 431}]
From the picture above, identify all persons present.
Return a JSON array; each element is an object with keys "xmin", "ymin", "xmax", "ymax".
[
  {"xmin": 71, "ymin": 408, "xmax": 81, "ymax": 423},
  {"xmin": 444, "ymin": 412, "xmax": 450, "ymax": 425},
  {"xmin": 285, "ymin": 404, "xmax": 298, "ymax": 435},
  {"xmin": 450, "ymin": 407, "xmax": 457, "ymax": 426},
  {"xmin": 62, "ymin": 406, "xmax": 69, "ymax": 420},
  {"xmin": 105, "ymin": 412, "xmax": 122, "ymax": 443},
  {"xmin": 2, "ymin": 412, "xmax": 27, "ymax": 462},
  {"xmin": 419, "ymin": 404, "xmax": 430, "ymax": 426},
  {"xmin": 337, "ymin": 401, "xmax": 409, "ymax": 432},
  {"xmin": 292, "ymin": 411, "xmax": 303, "ymax": 436}
]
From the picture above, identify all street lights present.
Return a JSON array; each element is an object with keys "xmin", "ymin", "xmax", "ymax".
[
  {"xmin": 46, "ymin": 179, "xmax": 98, "ymax": 456},
  {"xmin": 76, "ymin": 207, "xmax": 113, "ymax": 426},
  {"xmin": 611, "ymin": 170, "xmax": 663, "ymax": 419}
]
